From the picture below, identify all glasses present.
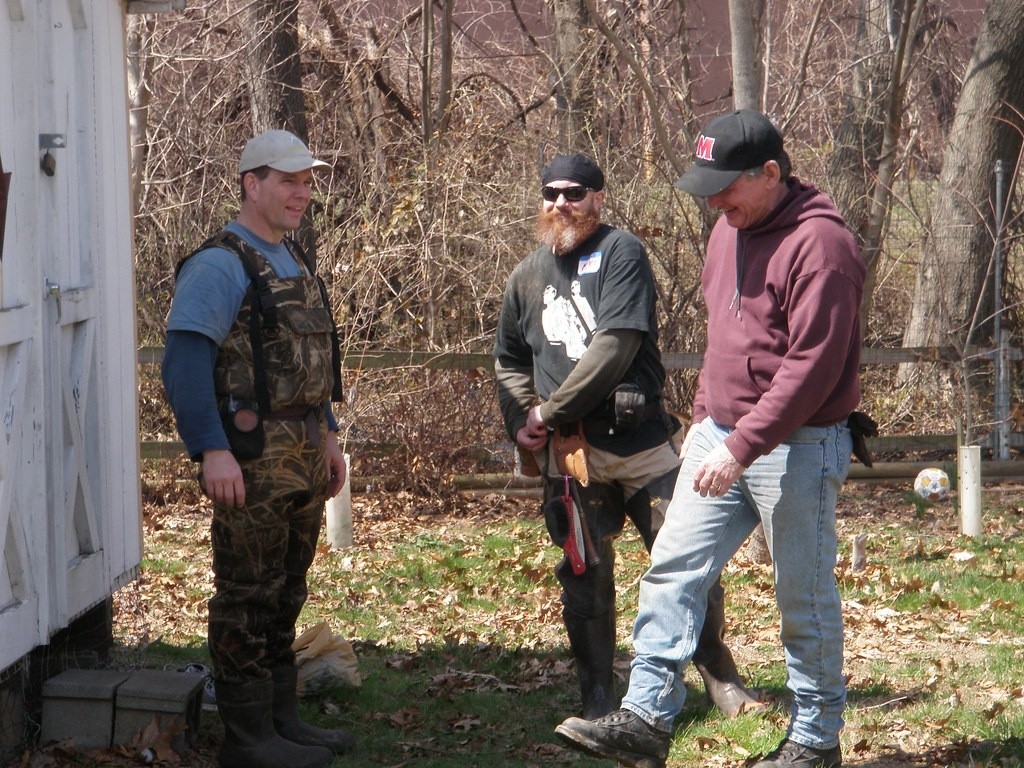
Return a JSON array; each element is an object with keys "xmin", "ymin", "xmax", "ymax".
[{"xmin": 540, "ymin": 186, "xmax": 596, "ymax": 201}]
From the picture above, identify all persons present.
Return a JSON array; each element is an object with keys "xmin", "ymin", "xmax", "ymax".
[
  {"xmin": 494, "ymin": 110, "xmax": 866, "ymax": 768},
  {"xmin": 161, "ymin": 131, "xmax": 356, "ymax": 768}
]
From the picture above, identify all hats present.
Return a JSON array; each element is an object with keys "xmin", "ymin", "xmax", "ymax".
[
  {"xmin": 542, "ymin": 154, "xmax": 605, "ymax": 191},
  {"xmin": 672, "ymin": 110, "xmax": 784, "ymax": 196},
  {"xmin": 239, "ymin": 130, "xmax": 334, "ymax": 174}
]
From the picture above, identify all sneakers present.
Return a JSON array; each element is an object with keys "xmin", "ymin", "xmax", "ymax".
[
  {"xmin": 554, "ymin": 709, "xmax": 671, "ymax": 768},
  {"xmin": 748, "ymin": 738, "xmax": 842, "ymax": 768}
]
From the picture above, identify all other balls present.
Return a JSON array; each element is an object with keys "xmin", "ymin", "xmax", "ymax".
[{"xmin": 912, "ymin": 467, "xmax": 951, "ymax": 503}]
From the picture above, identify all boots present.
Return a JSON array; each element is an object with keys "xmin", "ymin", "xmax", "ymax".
[
  {"xmin": 690, "ymin": 585, "xmax": 765, "ymax": 718},
  {"xmin": 210, "ymin": 669, "xmax": 334, "ymax": 768},
  {"xmin": 563, "ymin": 605, "xmax": 618, "ymax": 720},
  {"xmin": 270, "ymin": 666, "xmax": 354, "ymax": 753}
]
